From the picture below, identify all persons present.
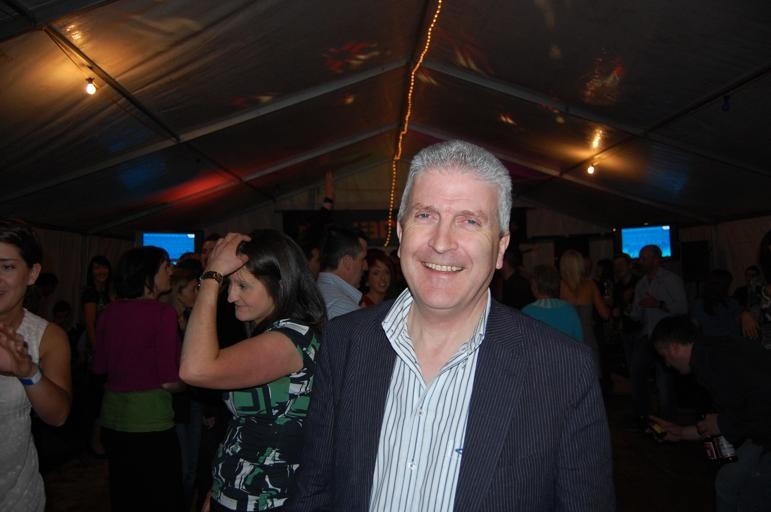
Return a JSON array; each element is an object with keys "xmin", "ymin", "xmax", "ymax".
[
  {"xmin": 24, "ymin": 225, "xmax": 408, "ymax": 510},
  {"xmin": 280, "ymin": 136, "xmax": 619, "ymax": 510},
  {"xmin": 293, "ymin": 166, "xmax": 338, "ymax": 249},
  {"xmin": 0, "ymin": 215, "xmax": 79, "ymax": 512},
  {"xmin": 501, "ymin": 246, "xmax": 771, "ymax": 511}
]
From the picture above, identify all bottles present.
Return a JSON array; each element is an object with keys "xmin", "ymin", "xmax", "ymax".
[
  {"xmin": 696, "ymin": 411, "xmax": 738, "ymax": 465},
  {"xmin": 641, "ymin": 415, "xmax": 664, "ymax": 439}
]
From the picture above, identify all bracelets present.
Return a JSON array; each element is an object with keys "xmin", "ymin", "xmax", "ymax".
[{"xmin": 17, "ymin": 366, "xmax": 44, "ymax": 386}]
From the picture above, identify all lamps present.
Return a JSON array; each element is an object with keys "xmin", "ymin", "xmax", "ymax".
[{"xmin": 82, "ymin": 76, "xmax": 99, "ymax": 97}]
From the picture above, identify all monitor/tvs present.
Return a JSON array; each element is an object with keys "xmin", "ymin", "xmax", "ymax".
[
  {"xmin": 141, "ymin": 230, "xmax": 199, "ymax": 266},
  {"xmin": 618, "ymin": 222, "xmax": 675, "ymax": 263}
]
